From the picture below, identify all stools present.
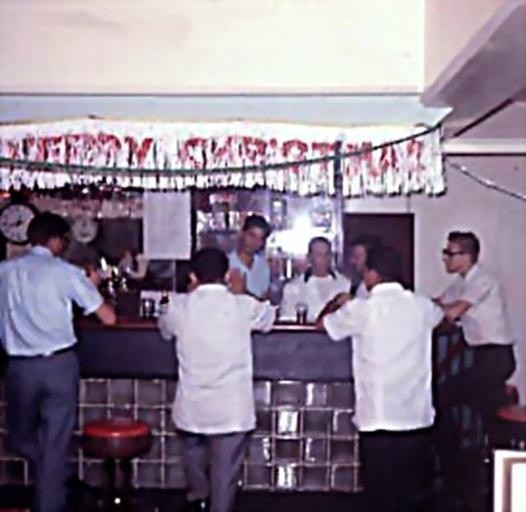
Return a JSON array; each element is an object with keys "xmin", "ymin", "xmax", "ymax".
[
  {"xmin": 469, "ymin": 383, "xmax": 526, "ymax": 455},
  {"xmin": 83, "ymin": 418, "xmax": 152, "ymax": 510}
]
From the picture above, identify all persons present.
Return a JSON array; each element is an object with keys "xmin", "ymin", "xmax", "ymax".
[
  {"xmin": 346, "ymin": 229, "xmax": 397, "ymax": 304},
  {"xmin": 431, "ymin": 227, "xmax": 518, "ymax": 448},
  {"xmin": 311, "ymin": 246, "xmax": 450, "ymax": 511},
  {"xmin": 157, "ymin": 243, "xmax": 279, "ymax": 510},
  {"xmin": 1, "ymin": 210, "xmax": 118, "ymax": 511},
  {"xmin": 220, "ymin": 214, "xmax": 274, "ymax": 305},
  {"xmin": 280, "ymin": 235, "xmax": 355, "ymax": 324}
]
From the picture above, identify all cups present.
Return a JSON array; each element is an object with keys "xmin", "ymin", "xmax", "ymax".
[
  {"xmin": 139, "ymin": 299, "xmax": 155, "ymax": 318},
  {"xmin": 295, "ymin": 303, "xmax": 309, "ymax": 326}
]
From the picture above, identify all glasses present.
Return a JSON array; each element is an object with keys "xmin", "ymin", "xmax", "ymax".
[{"xmin": 442, "ymin": 248, "xmax": 465, "ymax": 257}]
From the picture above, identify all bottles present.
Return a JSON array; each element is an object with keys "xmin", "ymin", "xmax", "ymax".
[{"xmin": 160, "ymin": 290, "xmax": 170, "ymax": 317}]
